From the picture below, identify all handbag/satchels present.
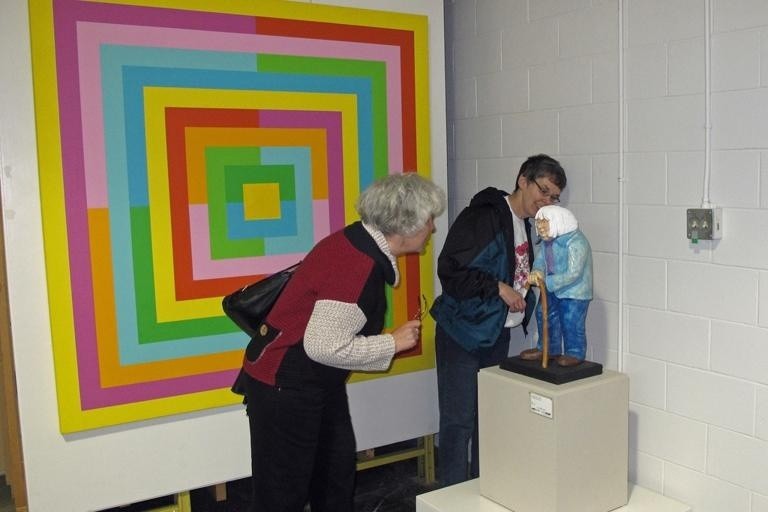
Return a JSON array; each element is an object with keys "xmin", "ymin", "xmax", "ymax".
[{"xmin": 221, "ymin": 261, "xmax": 303, "ymax": 339}]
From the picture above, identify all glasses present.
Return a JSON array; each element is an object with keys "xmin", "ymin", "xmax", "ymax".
[
  {"xmin": 533, "ymin": 179, "xmax": 560, "ymax": 203},
  {"xmin": 412, "ymin": 294, "xmax": 428, "ymax": 321}
]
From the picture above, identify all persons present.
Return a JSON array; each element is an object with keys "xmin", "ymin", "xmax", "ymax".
[
  {"xmin": 228, "ymin": 169, "xmax": 450, "ymax": 512},
  {"xmin": 517, "ymin": 203, "xmax": 594, "ymax": 369},
  {"xmin": 423, "ymin": 152, "xmax": 569, "ymax": 488}
]
